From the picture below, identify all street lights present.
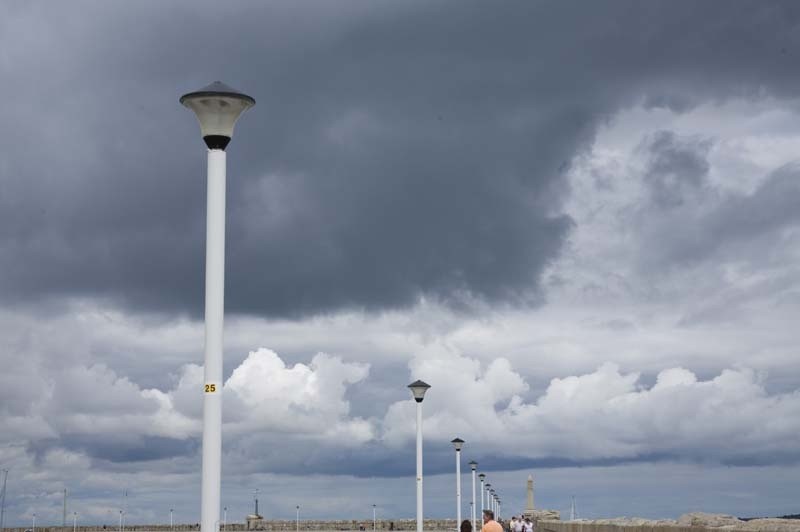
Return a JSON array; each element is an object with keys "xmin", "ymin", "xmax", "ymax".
[
  {"xmin": 449, "ymin": 435, "xmax": 466, "ymax": 532},
  {"xmin": 468, "ymin": 459, "xmax": 478, "ymax": 532},
  {"xmin": 477, "ymin": 472, "xmax": 487, "ymax": 528},
  {"xmin": 405, "ymin": 378, "xmax": 432, "ymax": 531},
  {"xmin": 28, "ymin": 487, "xmax": 380, "ymax": 531},
  {"xmin": 485, "ymin": 483, "xmax": 502, "ymax": 522},
  {"xmin": 179, "ymin": 77, "xmax": 257, "ymax": 532}
]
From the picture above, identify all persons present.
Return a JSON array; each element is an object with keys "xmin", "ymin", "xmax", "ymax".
[
  {"xmin": 480, "ymin": 509, "xmax": 505, "ymax": 531},
  {"xmin": 509, "ymin": 515, "xmax": 534, "ymax": 532}
]
